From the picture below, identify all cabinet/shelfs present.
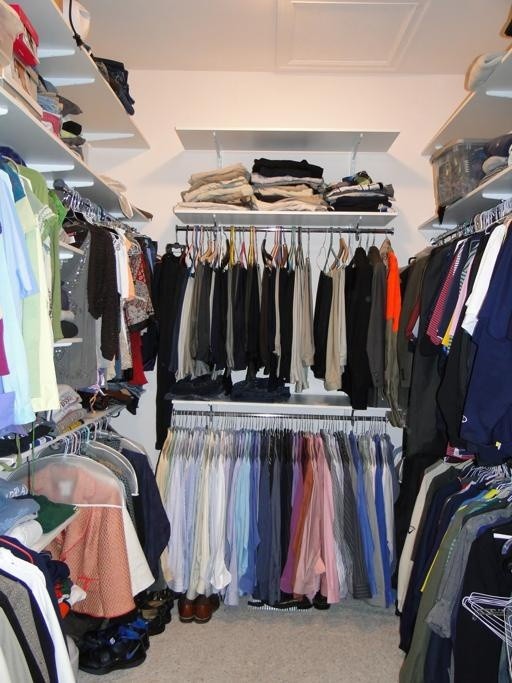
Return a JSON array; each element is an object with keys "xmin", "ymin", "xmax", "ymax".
[
  {"xmin": 1, "ymin": 0, "xmax": 153, "ymax": 469},
  {"xmin": 419, "ymin": 54, "xmax": 512, "ymax": 230},
  {"xmin": 173, "ymin": 126, "xmax": 401, "ymax": 412}
]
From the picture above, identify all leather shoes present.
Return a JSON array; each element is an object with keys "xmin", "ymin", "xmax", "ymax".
[
  {"xmin": 135, "ymin": 599, "xmax": 171, "ymax": 624},
  {"xmin": 178, "ymin": 594, "xmax": 193, "ymax": 623},
  {"xmin": 193, "ymin": 592, "xmax": 221, "ymax": 624},
  {"xmin": 107, "ymin": 607, "xmax": 165, "ymax": 635}
]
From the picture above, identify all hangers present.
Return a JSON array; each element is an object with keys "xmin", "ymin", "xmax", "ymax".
[
  {"xmin": 462, "ymin": 591, "xmax": 512, "ymax": 654},
  {"xmin": 56, "ymin": 177, "xmax": 139, "ymax": 238},
  {"xmin": 431, "ymin": 197, "xmax": 512, "ymax": 244},
  {"xmin": 455, "ymin": 448, "xmax": 512, "ymax": 505},
  {"xmin": 175, "ymin": 225, "xmax": 396, "ymax": 270},
  {"xmin": 163, "ymin": 408, "xmax": 393, "ymax": 467},
  {"xmin": 34, "ymin": 414, "xmax": 119, "ymax": 461}
]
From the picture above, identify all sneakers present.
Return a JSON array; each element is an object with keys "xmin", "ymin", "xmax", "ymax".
[
  {"xmin": 78, "ymin": 617, "xmax": 150, "ymax": 650},
  {"xmin": 79, "ymin": 625, "xmax": 147, "ymax": 675}
]
